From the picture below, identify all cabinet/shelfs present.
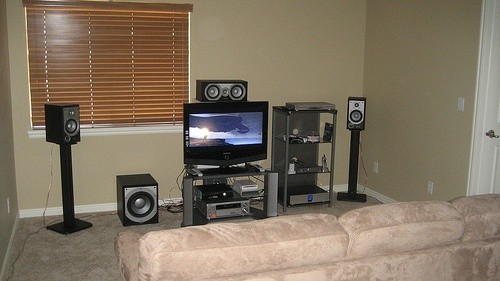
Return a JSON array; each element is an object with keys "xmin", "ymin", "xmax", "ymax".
[
  {"xmin": 183, "ymin": 172, "xmax": 278, "ymax": 227},
  {"xmin": 271, "ymin": 106, "xmax": 338, "ymax": 213}
]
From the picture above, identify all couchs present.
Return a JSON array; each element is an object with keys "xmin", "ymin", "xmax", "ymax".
[{"xmin": 113, "ymin": 193, "xmax": 500, "ymax": 281}]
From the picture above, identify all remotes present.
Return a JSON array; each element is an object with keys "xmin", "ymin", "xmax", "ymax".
[
  {"xmin": 247, "ymin": 163, "xmax": 265, "ymax": 173},
  {"xmin": 187, "ymin": 169, "xmax": 203, "ymax": 177}
]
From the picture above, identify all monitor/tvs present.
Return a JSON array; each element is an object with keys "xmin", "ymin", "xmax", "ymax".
[{"xmin": 183, "ymin": 101, "xmax": 269, "ymax": 175}]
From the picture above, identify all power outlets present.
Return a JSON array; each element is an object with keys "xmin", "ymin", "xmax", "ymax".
[
  {"xmin": 373, "ymin": 162, "xmax": 378, "ymax": 173},
  {"xmin": 427, "ymin": 181, "xmax": 433, "ymax": 193}
]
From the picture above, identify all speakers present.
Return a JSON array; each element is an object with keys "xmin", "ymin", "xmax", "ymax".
[
  {"xmin": 196, "ymin": 80, "xmax": 248, "ymax": 102},
  {"xmin": 45, "ymin": 103, "xmax": 80, "ymax": 144},
  {"xmin": 347, "ymin": 97, "xmax": 367, "ymax": 131},
  {"xmin": 116, "ymin": 173, "xmax": 159, "ymax": 226}
]
